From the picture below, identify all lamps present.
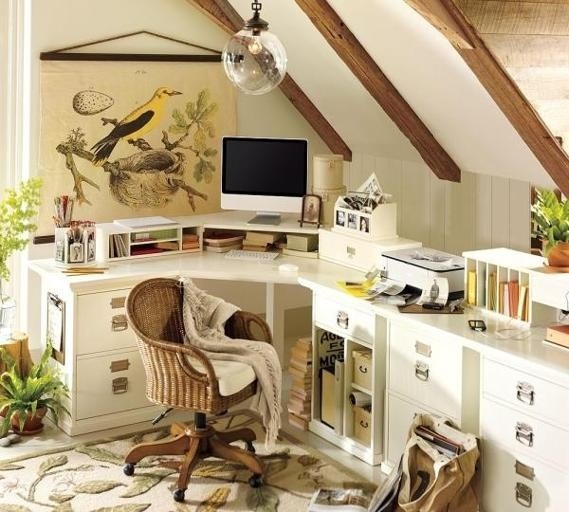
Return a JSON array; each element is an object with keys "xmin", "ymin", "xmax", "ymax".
[{"xmin": 221, "ymin": 1, "xmax": 287, "ymax": 96}]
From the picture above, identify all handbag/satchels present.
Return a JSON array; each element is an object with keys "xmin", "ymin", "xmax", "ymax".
[{"xmin": 395, "ymin": 411, "xmax": 481, "ymax": 512}]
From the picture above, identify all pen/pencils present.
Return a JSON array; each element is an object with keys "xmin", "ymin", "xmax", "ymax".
[{"xmin": 62, "ymin": 266, "xmax": 109, "ymax": 276}]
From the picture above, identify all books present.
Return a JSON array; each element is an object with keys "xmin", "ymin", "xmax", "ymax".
[
  {"xmin": 467, "ymin": 268, "xmax": 529, "ymax": 324},
  {"xmin": 370, "ymin": 284, "xmax": 422, "ymax": 306},
  {"xmin": 107, "ymin": 228, "xmax": 321, "ymax": 259},
  {"xmin": 285, "ymin": 337, "xmax": 312, "ymax": 432}
]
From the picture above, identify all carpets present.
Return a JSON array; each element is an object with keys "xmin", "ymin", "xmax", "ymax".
[{"xmin": 0, "ymin": 409, "xmax": 378, "ymax": 511}]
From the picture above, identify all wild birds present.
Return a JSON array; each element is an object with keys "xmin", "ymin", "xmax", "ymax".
[{"xmin": 89, "ymin": 87, "xmax": 183, "ymax": 168}]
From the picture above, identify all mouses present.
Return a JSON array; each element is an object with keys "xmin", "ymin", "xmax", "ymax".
[{"xmin": 279, "ymin": 263, "xmax": 300, "ymax": 273}]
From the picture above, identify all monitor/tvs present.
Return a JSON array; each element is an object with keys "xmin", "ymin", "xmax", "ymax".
[{"xmin": 220, "ymin": 136, "xmax": 310, "ymax": 227}]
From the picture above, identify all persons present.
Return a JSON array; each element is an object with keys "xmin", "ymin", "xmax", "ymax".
[
  {"xmin": 359, "ymin": 218, "xmax": 368, "ymax": 233},
  {"xmin": 336, "ymin": 211, "xmax": 345, "ymax": 227}
]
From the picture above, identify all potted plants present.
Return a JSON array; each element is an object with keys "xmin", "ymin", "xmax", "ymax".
[
  {"xmin": 530, "ymin": 185, "xmax": 569, "ymax": 267},
  {"xmin": 0, "ymin": 338, "xmax": 70, "ymax": 435}
]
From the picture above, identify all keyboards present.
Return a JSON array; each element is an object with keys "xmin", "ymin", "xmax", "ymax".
[{"xmin": 224, "ymin": 249, "xmax": 280, "ymax": 262}]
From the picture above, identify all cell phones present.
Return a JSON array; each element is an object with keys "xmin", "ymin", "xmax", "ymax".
[{"xmin": 468, "ymin": 320, "xmax": 486, "ymax": 331}]
